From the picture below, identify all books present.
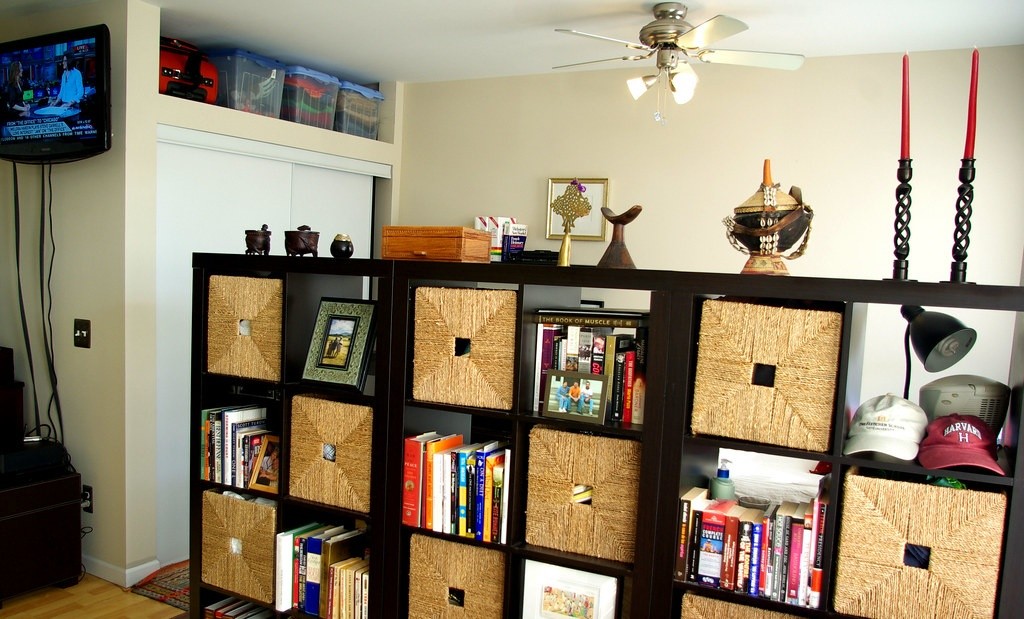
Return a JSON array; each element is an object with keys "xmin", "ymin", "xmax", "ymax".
[
  {"xmin": 401, "ymin": 431, "xmax": 512, "ymax": 544},
  {"xmin": 473, "ymin": 216, "xmax": 528, "ymax": 263},
  {"xmin": 673, "ymin": 477, "xmax": 830, "ymax": 609},
  {"xmin": 275, "ymin": 521, "xmax": 369, "ymax": 619},
  {"xmin": 530, "ymin": 306, "xmax": 646, "ymax": 425},
  {"xmin": 200, "ymin": 406, "xmax": 276, "ymax": 489}
]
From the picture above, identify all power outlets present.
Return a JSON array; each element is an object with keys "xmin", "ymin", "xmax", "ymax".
[{"xmin": 82, "ymin": 484, "xmax": 93, "ymax": 515}]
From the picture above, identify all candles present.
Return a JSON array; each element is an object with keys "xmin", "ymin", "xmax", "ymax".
[
  {"xmin": 899, "ymin": 51, "xmax": 911, "ymax": 161},
  {"xmin": 964, "ymin": 45, "xmax": 980, "ymax": 159}
]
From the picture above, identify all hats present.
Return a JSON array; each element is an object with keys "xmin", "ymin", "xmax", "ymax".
[
  {"xmin": 843, "ymin": 392, "xmax": 928, "ymax": 461},
  {"xmin": 917, "ymin": 413, "xmax": 1006, "ymax": 475}
]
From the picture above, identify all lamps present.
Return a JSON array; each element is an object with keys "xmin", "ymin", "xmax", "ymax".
[
  {"xmin": 625, "ymin": 2, "xmax": 699, "ymax": 105},
  {"xmin": 872, "ymin": 307, "xmax": 978, "ymax": 463}
]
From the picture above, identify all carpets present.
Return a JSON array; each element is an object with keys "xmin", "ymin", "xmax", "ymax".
[{"xmin": 131, "ymin": 558, "xmax": 190, "ymax": 611}]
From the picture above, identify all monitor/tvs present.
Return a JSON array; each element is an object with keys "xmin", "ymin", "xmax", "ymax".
[{"xmin": 0, "ymin": 24, "xmax": 112, "ymax": 165}]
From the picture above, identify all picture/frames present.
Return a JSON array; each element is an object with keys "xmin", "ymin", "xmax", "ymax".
[
  {"xmin": 248, "ymin": 435, "xmax": 280, "ymax": 495},
  {"xmin": 545, "ymin": 177, "xmax": 610, "ymax": 241},
  {"xmin": 542, "ymin": 369, "xmax": 608, "ymax": 426},
  {"xmin": 301, "ymin": 297, "xmax": 379, "ymax": 392},
  {"xmin": 523, "ymin": 558, "xmax": 618, "ymax": 618}
]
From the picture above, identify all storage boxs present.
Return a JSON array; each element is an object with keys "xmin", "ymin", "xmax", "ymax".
[
  {"xmin": 211, "ymin": 49, "xmax": 385, "ymax": 141},
  {"xmin": 382, "ymin": 225, "xmax": 491, "ymax": 262}
]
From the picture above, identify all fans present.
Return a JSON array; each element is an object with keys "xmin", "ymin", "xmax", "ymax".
[{"xmin": 549, "ymin": 14, "xmax": 808, "ymax": 106}]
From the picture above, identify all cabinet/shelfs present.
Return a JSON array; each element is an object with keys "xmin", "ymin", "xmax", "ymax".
[{"xmin": 186, "ymin": 252, "xmax": 1024, "ymax": 619}]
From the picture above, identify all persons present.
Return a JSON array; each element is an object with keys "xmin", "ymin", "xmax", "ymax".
[
  {"xmin": 257, "ymin": 447, "xmax": 279, "ymax": 486},
  {"xmin": 2, "ymin": 60, "xmax": 31, "ymax": 121},
  {"xmin": 577, "ymin": 381, "xmax": 593, "ymax": 415},
  {"xmin": 566, "ymin": 381, "xmax": 582, "ymax": 413},
  {"xmin": 556, "ymin": 380, "xmax": 570, "ymax": 412},
  {"xmin": 326, "ymin": 337, "xmax": 343, "ymax": 358},
  {"xmin": 47, "ymin": 50, "xmax": 83, "ymax": 121}
]
